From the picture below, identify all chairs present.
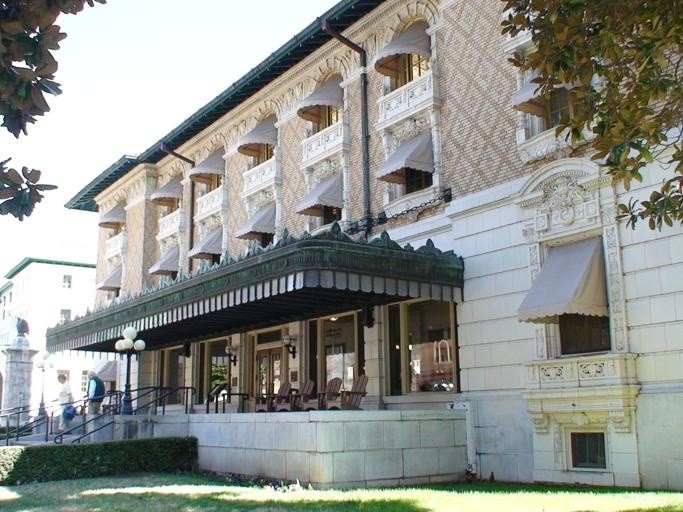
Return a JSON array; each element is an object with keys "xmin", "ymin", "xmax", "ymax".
[
  {"xmin": 274, "ymin": 380, "xmax": 315, "ymax": 412},
  {"xmin": 299, "ymin": 377, "xmax": 341, "ymax": 410},
  {"xmin": 325, "ymin": 375, "xmax": 368, "ymax": 410},
  {"xmin": 252, "ymin": 383, "xmax": 291, "ymax": 414}
]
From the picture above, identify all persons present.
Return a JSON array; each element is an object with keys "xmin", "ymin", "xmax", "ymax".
[
  {"xmin": 56, "ymin": 373, "xmax": 73, "ymax": 432},
  {"xmin": 85, "ymin": 370, "xmax": 105, "ymax": 415}
]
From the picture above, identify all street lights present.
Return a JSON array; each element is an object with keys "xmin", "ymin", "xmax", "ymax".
[
  {"xmin": 34, "ymin": 350, "xmax": 55, "ymax": 416},
  {"xmin": 114, "ymin": 326, "xmax": 145, "ymax": 414}
]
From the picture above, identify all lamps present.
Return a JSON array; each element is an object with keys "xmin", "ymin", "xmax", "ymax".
[
  {"xmin": 224, "ymin": 346, "xmax": 236, "ymax": 367},
  {"xmin": 282, "ymin": 334, "xmax": 295, "ymax": 359}
]
